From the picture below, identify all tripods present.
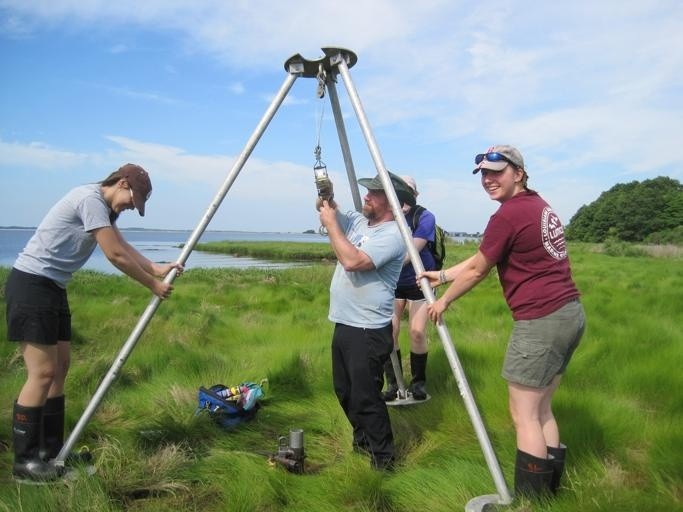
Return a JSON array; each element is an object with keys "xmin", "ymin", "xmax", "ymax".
[{"xmin": 52, "ymin": 72, "xmax": 518, "ymax": 507}]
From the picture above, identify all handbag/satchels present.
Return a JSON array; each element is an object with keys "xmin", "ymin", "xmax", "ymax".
[
  {"xmin": 194, "ymin": 384, "xmax": 260, "ymax": 433},
  {"xmin": 411, "ymin": 205, "xmax": 445, "ymax": 271}
]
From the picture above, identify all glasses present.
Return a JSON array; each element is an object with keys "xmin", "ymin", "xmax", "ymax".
[{"xmin": 475, "ymin": 152, "xmax": 525, "ymax": 175}]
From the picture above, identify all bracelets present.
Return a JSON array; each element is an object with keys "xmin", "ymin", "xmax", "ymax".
[{"xmin": 438, "ymin": 270, "xmax": 447, "ymax": 285}]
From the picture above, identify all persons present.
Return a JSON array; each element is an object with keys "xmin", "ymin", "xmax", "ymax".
[
  {"xmin": 415, "ymin": 144, "xmax": 585, "ymax": 510},
  {"xmin": 5, "ymin": 162, "xmax": 183, "ymax": 483},
  {"xmin": 382, "ymin": 176, "xmax": 438, "ymax": 402},
  {"xmin": 315, "ymin": 169, "xmax": 411, "ymax": 471}
]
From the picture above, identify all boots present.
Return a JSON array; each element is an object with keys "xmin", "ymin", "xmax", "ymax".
[
  {"xmin": 381, "ymin": 349, "xmax": 403, "ymax": 401},
  {"xmin": 39, "ymin": 395, "xmax": 94, "ymax": 466},
  {"xmin": 482, "ymin": 442, "xmax": 567, "ymax": 511},
  {"xmin": 12, "ymin": 398, "xmax": 67, "ymax": 485},
  {"xmin": 411, "ymin": 350, "xmax": 428, "ymax": 400}
]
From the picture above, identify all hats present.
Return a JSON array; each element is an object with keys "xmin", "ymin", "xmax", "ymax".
[
  {"xmin": 357, "ymin": 170, "xmax": 419, "ymax": 209},
  {"xmin": 473, "ymin": 144, "xmax": 524, "ymax": 174},
  {"xmin": 118, "ymin": 163, "xmax": 152, "ymax": 216}
]
